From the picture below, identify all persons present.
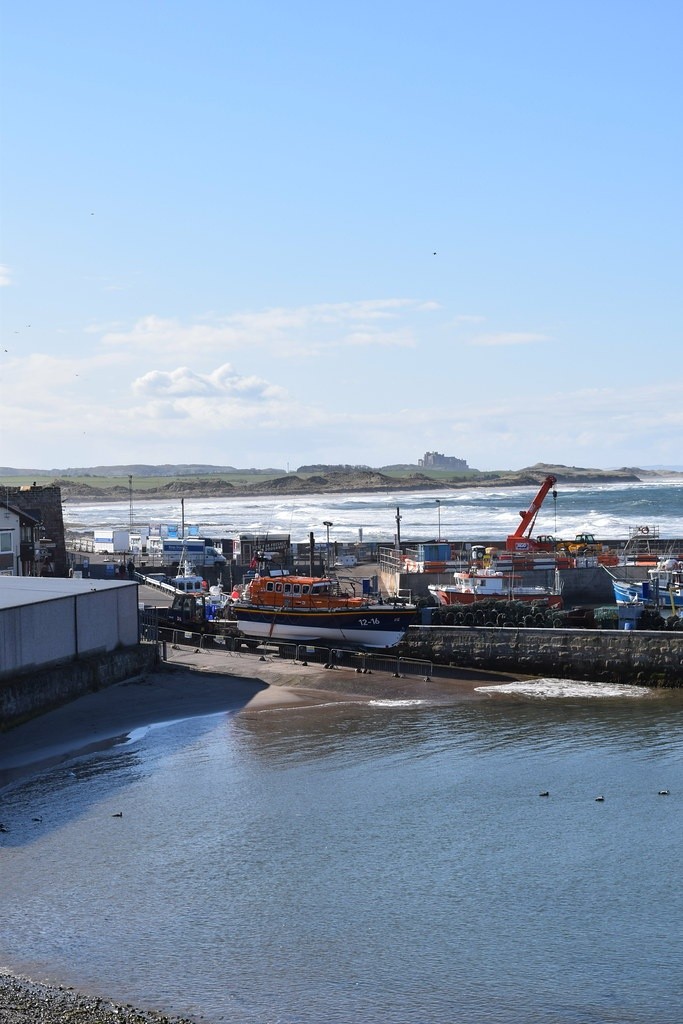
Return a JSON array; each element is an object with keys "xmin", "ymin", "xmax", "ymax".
[
  {"xmin": 432, "ymin": 598, "xmax": 563, "ymax": 628},
  {"xmin": 583, "ymin": 611, "xmax": 597, "ymax": 629},
  {"xmin": 201, "ymin": 579, "xmax": 207, "ymax": 591},
  {"xmin": 601, "ymin": 614, "xmax": 618, "ymax": 629},
  {"xmin": 378, "ymin": 597, "xmax": 391, "ymax": 605},
  {"xmin": 119, "ymin": 562, "xmax": 126, "ymax": 580},
  {"xmin": 127, "ymin": 560, "xmax": 135, "ymax": 580},
  {"xmin": 393, "ymin": 600, "xmax": 397, "ymax": 606},
  {"xmin": 636, "ymin": 605, "xmax": 683, "ymax": 631},
  {"xmin": 402, "ymin": 600, "xmax": 406, "ymax": 606},
  {"xmin": 231, "ymin": 584, "xmax": 243, "ymax": 600}
]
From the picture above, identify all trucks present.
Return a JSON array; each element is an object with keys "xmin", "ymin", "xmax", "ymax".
[{"xmin": 161, "ymin": 538, "xmax": 228, "ymax": 567}]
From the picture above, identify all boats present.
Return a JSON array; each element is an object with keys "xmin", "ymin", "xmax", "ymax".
[
  {"xmin": 609, "ymin": 556, "xmax": 683, "ymax": 608},
  {"xmin": 169, "ymin": 537, "xmax": 205, "ymax": 596},
  {"xmin": 230, "ymin": 574, "xmax": 418, "ymax": 650},
  {"xmin": 427, "ymin": 564, "xmax": 567, "ymax": 609}
]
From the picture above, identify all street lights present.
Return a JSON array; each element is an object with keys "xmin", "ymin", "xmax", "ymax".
[
  {"xmin": 435, "ymin": 499, "xmax": 441, "ymax": 542},
  {"xmin": 323, "ymin": 520, "xmax": 333, "ymax": 571}
]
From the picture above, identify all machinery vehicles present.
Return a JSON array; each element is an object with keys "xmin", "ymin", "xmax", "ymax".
[
  {"xmin": 500, "ymin": 476, "xmax": 558, "ymax": 552},
  {"xmin": 569, "ymin": 532, "xmax": 602, "ymax": 555},
  {"xmin": 535, "ymin": 534, "xmax": 557, "ymax": 554}
]
